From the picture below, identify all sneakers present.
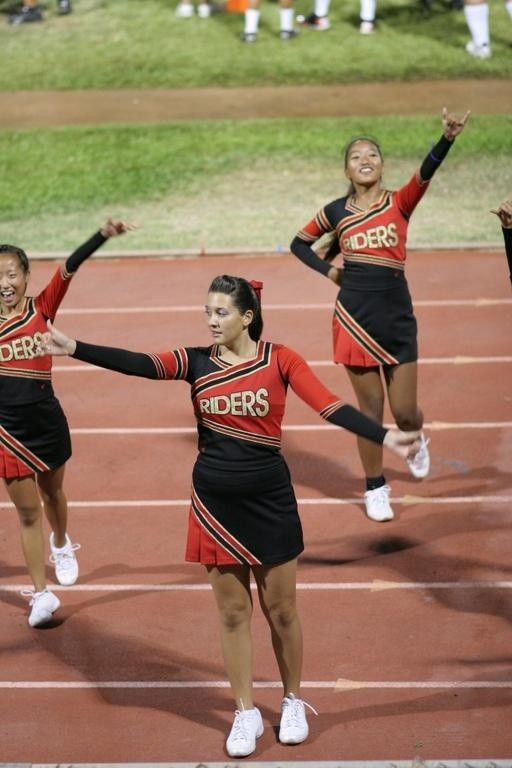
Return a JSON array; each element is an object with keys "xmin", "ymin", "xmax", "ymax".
[
  {"xmin": 48, "ymin": 532, "xmax": 81, "ymax": 586},
  {"xmin": 464, "ymin": 40, "xmax": 491, "ymax": 61},
  {"xmin": 20, "ymin": 588, "xmax": 61, "ymax": 628},
  {"xmin": 279, "ymin": 693, "xmax": 318, "ymax": 744},
  {"xmin": 364, "ymin": 484, "xmax": 395, "ymax": 522},
  {"xmin": 225, "ymin": 697, "xmax": 265, "ymax": 757},
  {"xmin": 406, "ymin": 433, "xmax": 431, "ymax": 480},
  {"xmin": 173, "ymin": 4, "xmax": 375, "ymax": 40},
  {"xmin": 9, "ymin": 5, "xmax": 43, "ymax": 26},
  {"xmin": 57, "ymin": 2, "xmax": 70, "ymax": 13}
]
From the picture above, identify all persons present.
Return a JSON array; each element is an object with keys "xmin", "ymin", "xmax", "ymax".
[
  {"xmin": 9, "ymin": 0, "xmax": 71, "ymax": 24},
  {"xmin": 491, "ymin": 199, "xmax": 512, "ymax": 286},
  {"xmin": 41, "ymin": 274, "xmax": 420, "ymax": 756},
  {"xmin": 290, "ymin": 103, "xmax": 472, "ymax": 522},
  {"xmin": 1, "ymin": 214, "xmax": 134, "ymax": 627}
]
